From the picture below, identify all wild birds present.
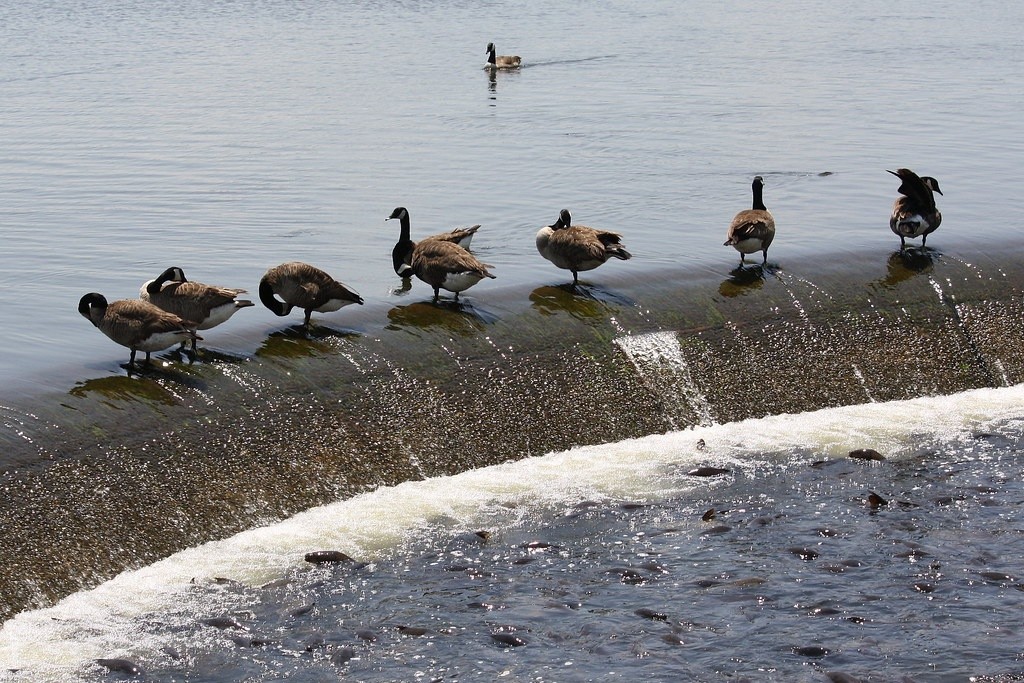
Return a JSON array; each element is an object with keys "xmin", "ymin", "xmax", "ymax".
[
  {"xmin": 384, "ymin": 207, "xmax": 481, "ymax": 278},
  {"xmin": 77, "ymin": 293, "xmax": 204, "ymax": 369},
  {"xmin": 259, "ymin": 262, "xmax": 364, "ymax": 339},
  {"xmin": 886, "ymin": 168, "xmax": 944, "ymax": 256},
  {"xmin": 411, "ymin": 240, "xmax": 496, "ymax": 302},
  {"xmin": 723, "ymin": 175, "xmax": 775, "ymax": 269},
  {"xmin": 139, "ymin": 266, "xmax": 255, "ymax": 357},
  {"xmin": 483, "ymin": 43, "xmax": 522, "ymax": 69},
  {"xmin": 536, "ymin": 209, "xmax": 633, "ymax": 285}
]
